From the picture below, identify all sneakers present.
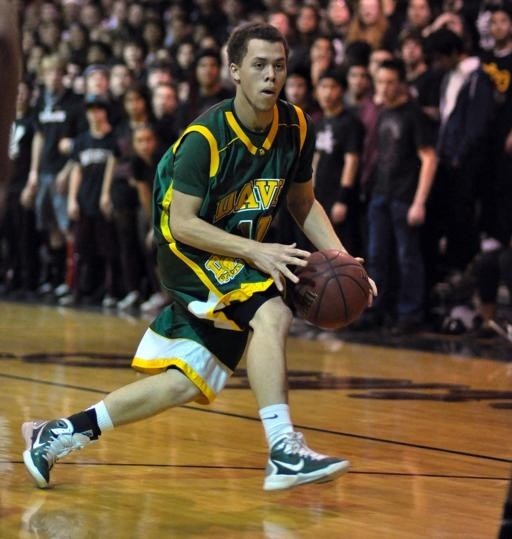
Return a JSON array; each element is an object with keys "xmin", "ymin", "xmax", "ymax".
[
  {"xmin": 21, "ymin": 418, "xmax": 74, "ymax": 489},
  {"xmin": 360, "ymin": 312, "xmax": 512, "ymax": 341},
  {"xmin": 262, "ymin": 432, "xmax": 350, "ymax": 492},
  {"xmin": 0, "ymin": 279, "xmax": 168, "ymax": 311}
]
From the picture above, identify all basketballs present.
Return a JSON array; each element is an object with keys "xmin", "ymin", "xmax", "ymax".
[{"xmin": 290, "ymin": 249, "xmax": 369, "ymax": 331}]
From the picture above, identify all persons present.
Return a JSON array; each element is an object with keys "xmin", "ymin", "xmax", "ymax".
[
  {"xmin": 0, "ymin": 0, "xmax": 512, "ymax": 344},
  {"xmin": 19, "ymin": 21, "xmax": 381, "ymax": 493}
]
